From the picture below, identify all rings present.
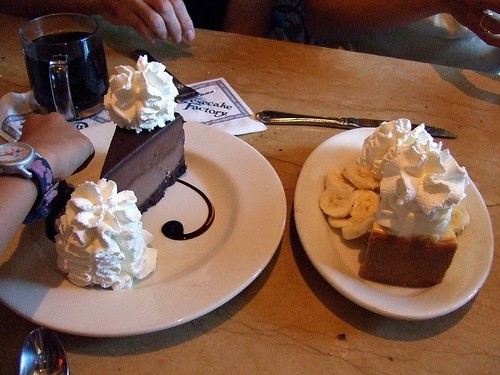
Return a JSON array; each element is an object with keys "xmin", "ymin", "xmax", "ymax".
[{"xmin": 479, "ymin": 13, "xmax": 491, "ymax": 32}]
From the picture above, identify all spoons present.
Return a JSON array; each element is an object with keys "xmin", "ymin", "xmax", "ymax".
[{"xmin": 19, "ymin": 326, "xmax": 70, "ymax": 375}]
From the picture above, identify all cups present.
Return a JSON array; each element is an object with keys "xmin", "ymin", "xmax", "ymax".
[{"xmin": 18, "ymin": 13, "xmax": 109, "ymax": 122}]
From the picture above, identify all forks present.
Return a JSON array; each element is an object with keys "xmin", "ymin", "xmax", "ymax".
[{"xmin": 131, "ymin": 48, "xmax": 215, "ymax": 104}]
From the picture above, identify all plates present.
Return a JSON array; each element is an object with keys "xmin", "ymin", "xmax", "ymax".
[
  {"xmin": 293, "ymin": 126, "xmax": 495, "ymax": 323},
  {"xmin": 0, "ymin": 120, "xmax": 288, "ymax": 339}
]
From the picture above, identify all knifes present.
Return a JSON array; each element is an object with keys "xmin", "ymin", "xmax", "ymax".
[{"xmin": 256, "ymin": 110, "xmax": 457, "ymax": 140}]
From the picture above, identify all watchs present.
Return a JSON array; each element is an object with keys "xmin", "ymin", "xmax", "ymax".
[{"xmin": 0, "ymin": 142, "xmax": 59, "ymax": 225}]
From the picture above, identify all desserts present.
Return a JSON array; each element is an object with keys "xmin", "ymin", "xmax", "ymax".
[
  {"xmin": 320, "ymin": 118, "xmax": 469, "ymax": 288},
  {"xmin": 54, "ymin": 55, "xmax": 187, "ymax": 291}
]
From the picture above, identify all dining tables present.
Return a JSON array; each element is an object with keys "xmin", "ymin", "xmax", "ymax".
[{"xmin": 0, "ymin": 11, "xmax": 500, "ymax": 375}]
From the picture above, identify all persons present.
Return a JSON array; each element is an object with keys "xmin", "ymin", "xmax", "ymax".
[
  {"xmin": 0, "ymin": 0, "xmax": 500, "ymax": 46},
  {"xmin": 0, "ymin": 111, "xmax": 96, "ymax": 268}
]
